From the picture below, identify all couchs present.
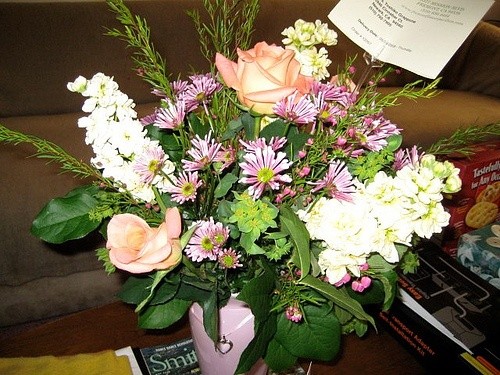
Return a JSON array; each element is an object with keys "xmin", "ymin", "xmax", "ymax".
[{"xmin": 0, "ymin": 0, "xmax": 500, "ymax": 327}]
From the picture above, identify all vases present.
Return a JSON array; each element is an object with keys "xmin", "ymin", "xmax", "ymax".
[{"xmin": 187, "ymin": 291, "xmax": 269, "ymax": 375}]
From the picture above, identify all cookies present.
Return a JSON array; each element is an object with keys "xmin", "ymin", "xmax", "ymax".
[
  {"xmin": 466, "ymin": 202, "xmax": 498, "ymax": 228},
  {"xmin": 476, "ymin": 181, "xmax": 500, "ymax": 203}
]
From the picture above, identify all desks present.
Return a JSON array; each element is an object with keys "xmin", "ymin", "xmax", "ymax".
[{"xmin": 0, "ymin": 302, "xmax": 430, "ymax": 375}]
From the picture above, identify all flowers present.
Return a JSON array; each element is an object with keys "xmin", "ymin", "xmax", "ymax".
[{"xmin": 0, "ymin": 0, "xmax": 500, "ymax": 375}]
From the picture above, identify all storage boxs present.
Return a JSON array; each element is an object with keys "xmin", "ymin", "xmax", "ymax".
[
  {"xmin": 368, "ymin": 238, "xmax": 500, "ymax": 375},
  {"xmin": 456, "ymin": 220, "xmax": 500, "ymax": 290},
  {"xmin": 436, "ymin": 140, "xmax": 500, "ymax": 259}
]
